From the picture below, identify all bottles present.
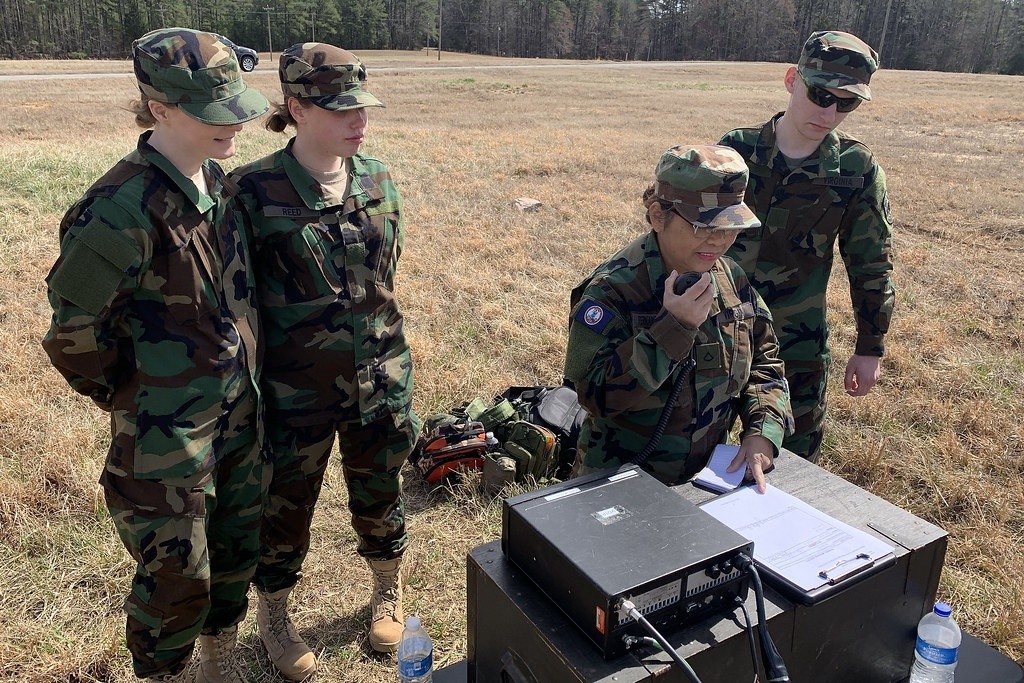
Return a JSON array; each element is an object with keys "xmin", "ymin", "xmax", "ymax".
[
  {"xmin": 397, "ymin": 617, "xmax": 433, "ymax": 683},
  {"xmin": 910, "ymin": 603, "xmax": 962, "ymax": 683}
]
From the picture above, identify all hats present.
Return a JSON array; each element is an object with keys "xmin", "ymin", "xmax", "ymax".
[
  {"xmin": 279, "ymin": 42, "xmax": 386, "ymax": 112},
  {"xmin": 654, "ymin": 144, "xmax": 761, "ymax": 230},
  {"xmin": 132, "ymin": 27, "xmax": 271, "ymax": 126},
  {"xmin": 798, "ymin": 31, "xmax": 880, "ymax": 101}
]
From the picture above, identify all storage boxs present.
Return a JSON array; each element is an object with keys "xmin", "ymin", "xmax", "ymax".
[{"xmin": 466, "ymin": 447, "xmax": 950, "ymax": 683}]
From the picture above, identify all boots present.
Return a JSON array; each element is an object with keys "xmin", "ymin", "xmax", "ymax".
[
  {"xmin": 196, "ymin": 624, "xmax": 248, "ymax": 683},
  {"xmin": 257, "ymin": 581, "xmax": 317, "ymax": 681},
  {"xmin": 365, "ymin": 555, "xmax": 405, "ymax": 652},
  {"xmin": 148, "ymin": 663, "xmax": 193, "ymax": 683}
]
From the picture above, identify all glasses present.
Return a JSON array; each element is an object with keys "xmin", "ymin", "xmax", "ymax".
[
  {"xmin": 664, "ymin": 205, "xmax": 743, "ymax": 237},
  {"xmin": 799, "ymin": 69, "xmax": 862, "ymax": 113}
]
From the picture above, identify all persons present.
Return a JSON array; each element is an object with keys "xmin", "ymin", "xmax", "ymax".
[
  {"xmin": 227, "ymin": 41, "xmax": 425, "ymax": 680},
  {"xmin": 41, "ymin": 26, "xmax": 274, "ymax": 683},
  {"xmin": 717, "ymin": 30, "xmax": 895, "ymax": 467},
  {"xmin": 563, "ymin": 144, "xmax": 796, "ymax": 494}
]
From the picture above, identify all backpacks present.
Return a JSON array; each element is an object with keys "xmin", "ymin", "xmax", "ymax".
[{"xmin": 407, "ymin": 386, "xmax": 588, "ymax": 495}]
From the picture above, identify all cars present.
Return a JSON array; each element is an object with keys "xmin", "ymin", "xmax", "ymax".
[{"xmin": 207, "ymin": 30, "xmax": 259, "ymax": 72}]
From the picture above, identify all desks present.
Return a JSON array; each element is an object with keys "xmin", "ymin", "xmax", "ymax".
[{"xmin": 432, "ymin": 630, "xmax": 1024, "ymax": 683}]
